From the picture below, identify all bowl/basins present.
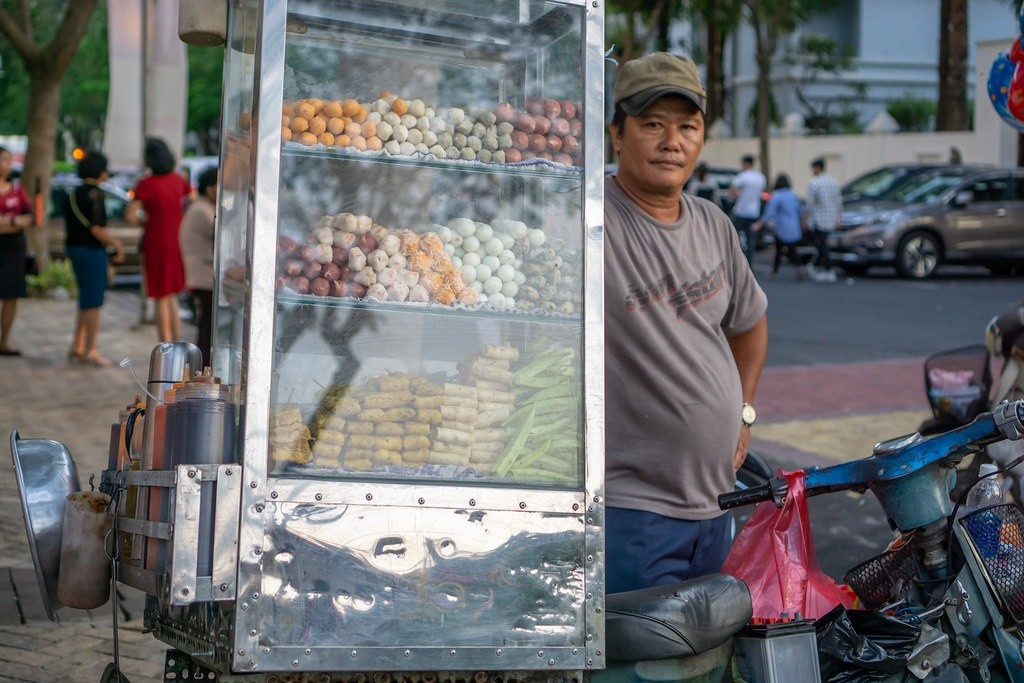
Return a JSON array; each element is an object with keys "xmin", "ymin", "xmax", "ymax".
[{"xmin": 10, "ymin": 430, "xmax": 82, "ymax": 621}]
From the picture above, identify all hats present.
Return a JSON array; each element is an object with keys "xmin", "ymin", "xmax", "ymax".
[{"xmin": 613, "ymin": 51, "xmax": 708, "ymax": 118}]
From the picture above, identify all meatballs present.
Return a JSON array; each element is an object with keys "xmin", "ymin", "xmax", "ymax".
[
  {"xmin": 276, "ymin": 211, "xmax": 583, "ymax": 318},
  {"xmin": 228, "ymin": 92, "xmax": 583, "ymax": 170}
]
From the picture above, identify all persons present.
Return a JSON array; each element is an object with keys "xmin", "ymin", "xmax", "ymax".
[
  {"xmin": 549, "ymin": 54, "xmax": 769, "ymax": 582},
  {"xmin": 690, "ymin": 152, "xmax": 843, "ymax": 281},
  {"xmin": 0, "ymin": 135, "xmax": 217, "ymax": 367}
]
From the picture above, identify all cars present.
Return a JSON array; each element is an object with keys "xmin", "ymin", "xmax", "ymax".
[
  {"xmin": 687, "ymin": 155, "xmax": 1024, "ymax": 283},
  {"xmin": 0, "ymin": 130, "xmax": 220, "ymax": 275}
]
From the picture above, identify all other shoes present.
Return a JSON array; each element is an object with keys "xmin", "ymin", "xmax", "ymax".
[
  {"xmin": 0, "ymin": 350, "xmax": 20, "ymax": 356},
  {"xmin": 84, "ymin": 354, "xmax": 112, "ymax": 366},
  {"xmin": 67, "ymin": 347, "xmax": 86, "ymax": 364}
]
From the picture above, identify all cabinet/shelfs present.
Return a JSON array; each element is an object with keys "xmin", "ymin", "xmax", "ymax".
[{"xmin": 143, "ymin": 0, "xmax": 605, "ymax": 675}]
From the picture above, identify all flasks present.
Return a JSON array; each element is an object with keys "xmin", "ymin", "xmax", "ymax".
[{"xmin": 128, "ymin": 342, "xmax": 205, "ymax": 561}]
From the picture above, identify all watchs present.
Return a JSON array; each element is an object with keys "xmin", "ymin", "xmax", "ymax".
[{"xmin": 742, "ymin": 403, "xmax": 755, "ymax": 426}]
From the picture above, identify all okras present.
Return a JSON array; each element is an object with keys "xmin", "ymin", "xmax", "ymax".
[{"xmin": 493, "ymin": 350, "xmax": 581, "ymax": 487}]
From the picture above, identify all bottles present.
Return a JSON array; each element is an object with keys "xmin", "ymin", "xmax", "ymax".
[
  {"xmin": 964, "ymin": 463, "xmax": 1003, "ymax": 572},
  {"xmin": 112, "ymin": 363, "xmax": 235, "ymax": 577}
]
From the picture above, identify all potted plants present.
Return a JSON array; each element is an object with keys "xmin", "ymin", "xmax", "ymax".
[{"xmin": 886, "ymin": 91, "xmax": 937, "ymax": 132}]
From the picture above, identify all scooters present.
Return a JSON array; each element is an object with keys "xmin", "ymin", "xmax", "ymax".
[{"xmin": 102, "ymin": 297, "xmax": 1023, "ymax": 682}]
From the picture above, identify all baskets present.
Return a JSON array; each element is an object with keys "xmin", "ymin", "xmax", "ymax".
[{"xmin": 842, "ymin": 504, "xmax": 1024, "ymax": 617}]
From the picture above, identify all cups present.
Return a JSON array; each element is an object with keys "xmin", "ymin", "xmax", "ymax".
[{"xmin": 57, "ymin": 488, "xmax": 114, "ymax": 609}]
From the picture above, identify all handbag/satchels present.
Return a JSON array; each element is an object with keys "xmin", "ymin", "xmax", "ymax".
[{"xmin": 720, "ymin": 467, "xmax": 857, "ymax": 625}]
